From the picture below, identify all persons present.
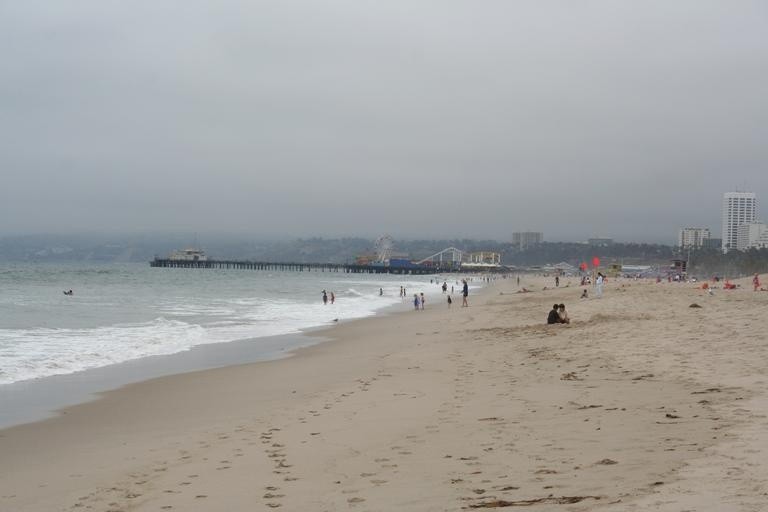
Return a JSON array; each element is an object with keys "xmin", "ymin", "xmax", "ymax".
[
  {"xmin": 322, "ymin": 292, "xmax": 327, "ymax": 304},
  {"xmin": 557, "ymin": 303, "xmax": 569, "ymax": 323},
  {"xmin": 547, "ymin": 304, "xmax": 566, "ymax": 324},
  {"xmin": 635, "ymin": 271, "xmax": 697, "ymax": 285},
  {"xmin": 330, "ymin": 292, "xmax": 334, "ymax": 304},
  {"xmin": 553, "ymin": 268, "xmax": 606, "ymax": 299},
  {"xmin": 706, "ymin": 270, "xmax": 760, "ymax": 296},
  {"xmin": 399, "ymin": 276, "xmax": 469, "ymax": 310}
]
